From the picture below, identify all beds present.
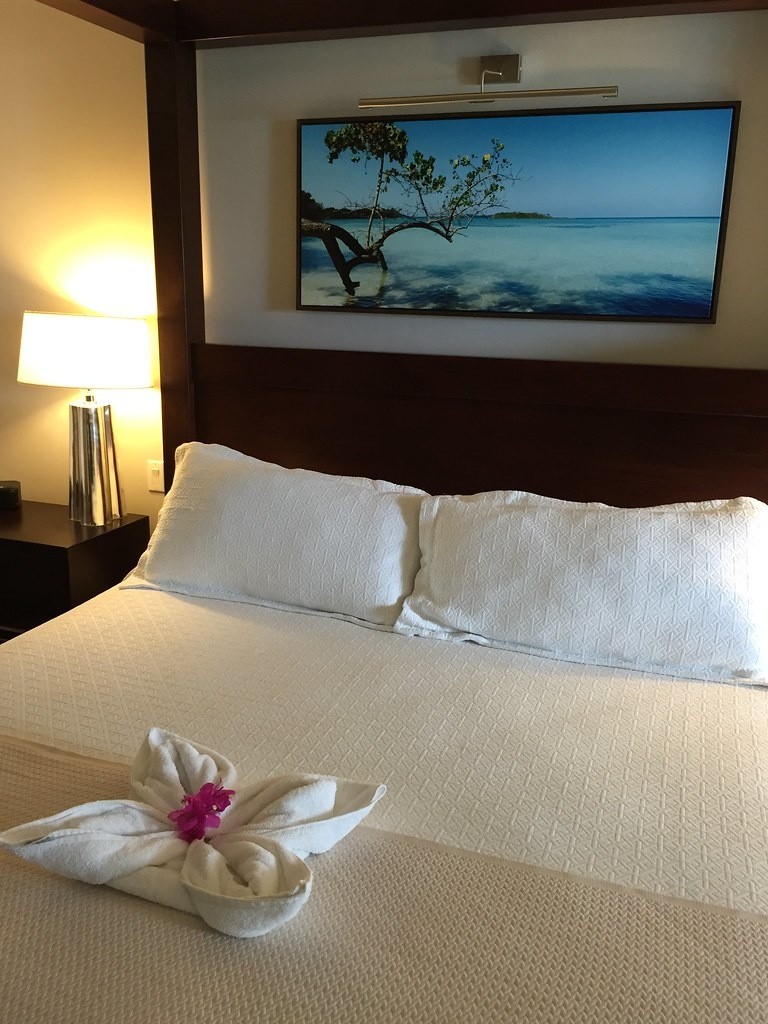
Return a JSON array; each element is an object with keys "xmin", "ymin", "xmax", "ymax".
[{"xmin": 0, "ymin": 343, "xmax": 768, "ymax": 1024}]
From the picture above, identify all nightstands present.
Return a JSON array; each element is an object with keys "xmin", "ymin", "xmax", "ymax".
[{"xmin": 0, "ymin": 499, "xmax": 151, "ymax": 645}]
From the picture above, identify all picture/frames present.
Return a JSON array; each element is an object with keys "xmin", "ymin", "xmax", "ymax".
[{"xmin": 295, "ymin": 100, "xmax": 742, "ymax": 324}]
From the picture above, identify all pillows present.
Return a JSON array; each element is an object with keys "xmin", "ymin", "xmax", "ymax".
[
  {"xmin": 118, "ymin": 442, "xmax": 432, "ymax": 633},
  {"xmin": 393, "ymin": 489, "xmax": 768, "ymax": 687}
]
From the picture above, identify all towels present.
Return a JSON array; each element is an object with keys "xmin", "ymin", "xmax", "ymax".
[{"xmin": 0, "ymin": 727, "xmax": 391, "ymax": 937}]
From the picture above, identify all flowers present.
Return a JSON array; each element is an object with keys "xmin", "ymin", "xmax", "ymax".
[{"xmin": 168, "ymin": 783, "xmax": 235, "ymax": 843}]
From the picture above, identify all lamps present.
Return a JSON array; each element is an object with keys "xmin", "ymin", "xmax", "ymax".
[
  {"xmin": 357, "ymin": 53, "xmax": 619, "ymax": 110},
  {"xmin": 17, "ymin": 311, "xmax": 152, "ymax": 528}
]
